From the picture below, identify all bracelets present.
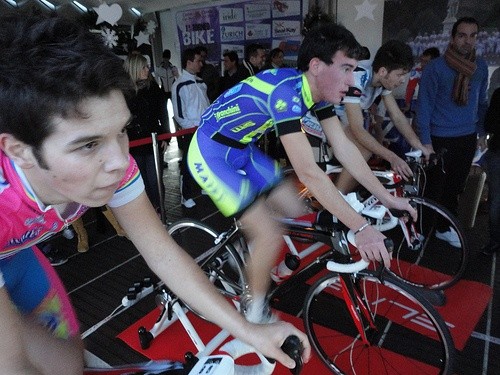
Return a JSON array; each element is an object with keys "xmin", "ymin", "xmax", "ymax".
[{"xmin": 353, "ymin": 222, "xmax": 372, "ymax": 234}]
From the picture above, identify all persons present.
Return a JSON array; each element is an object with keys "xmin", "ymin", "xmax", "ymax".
[
  {"xmin": 1, "ymin": 0, "xmax": 500, "ymax": 375},
  {"xmin": 416, "ymin": 16, "xmax": 489, "ymax": 213}
]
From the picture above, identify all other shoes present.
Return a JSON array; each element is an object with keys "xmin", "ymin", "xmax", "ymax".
[
  {"xmin": 482, "ymin": 240, "xmax": 500, "ymax": 255},
  {"xmin": 63, "ymin": 227, "xmax": 74, "ymax": 239}
]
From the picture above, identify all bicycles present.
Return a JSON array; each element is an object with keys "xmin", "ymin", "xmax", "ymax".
[
  {"xmin": 257, "ymin": 146, "xmax": 465, "ymax": 292},
  {"xmin": 84, "ymin": 336, "xmax": 305, "ymax": 375},
  {"xmin": 166, "ymin": 207, "xmax": 456, "ymax": 375}
]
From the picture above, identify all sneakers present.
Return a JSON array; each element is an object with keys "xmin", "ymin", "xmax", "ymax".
[
  {"xmin": 201, "ymin": 189, "xmax": 208, "ymax": 195},
  {"xmin": 435, "ymin": 226, "xmax": 463, "ymax": 248},
  {"xmin": 181, "ymin": 195, "xmax": 196, "ymax": 209}
]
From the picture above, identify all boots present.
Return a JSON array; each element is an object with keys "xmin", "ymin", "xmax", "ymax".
[
  {"xmin": 72, "ymin": 218, "xmax": 89, "ymax": 253},
  {"xmin": 102, "ymin": 205, "xmax": 126, "ymax": 237}
]
